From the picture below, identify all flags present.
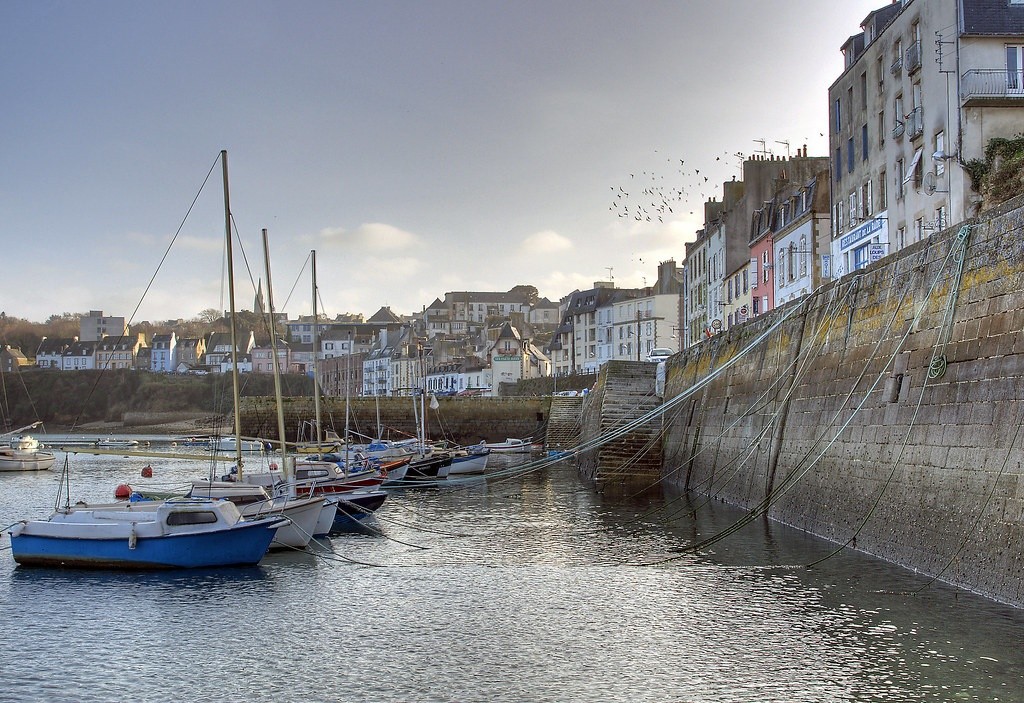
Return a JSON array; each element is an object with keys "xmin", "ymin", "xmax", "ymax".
[{"xmin": 430, "ymin": 395, "xmax": 439, "ymax": 409}]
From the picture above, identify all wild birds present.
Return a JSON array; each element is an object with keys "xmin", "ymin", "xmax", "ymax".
[{"xmin": 607, "ymin": 131, "xmax": 823, "ymax": 224}]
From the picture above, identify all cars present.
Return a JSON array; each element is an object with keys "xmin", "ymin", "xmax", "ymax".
[{"xmin": 645, "ymin": 347, "xmax": 675, "ymax": 363}]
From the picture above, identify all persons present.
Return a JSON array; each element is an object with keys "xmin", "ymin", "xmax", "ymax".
[{"xmin": 701, "ymin": 330, "xmax": 708, "ymax": 341}]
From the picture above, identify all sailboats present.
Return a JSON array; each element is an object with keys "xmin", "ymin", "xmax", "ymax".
[
  {"xmin": 0, "ymin": 337, "xmax": 57, "ymax": 471},
  {"xmin": 8, "ymin": 147, "xmax": 545, "ymax": 570}
]
